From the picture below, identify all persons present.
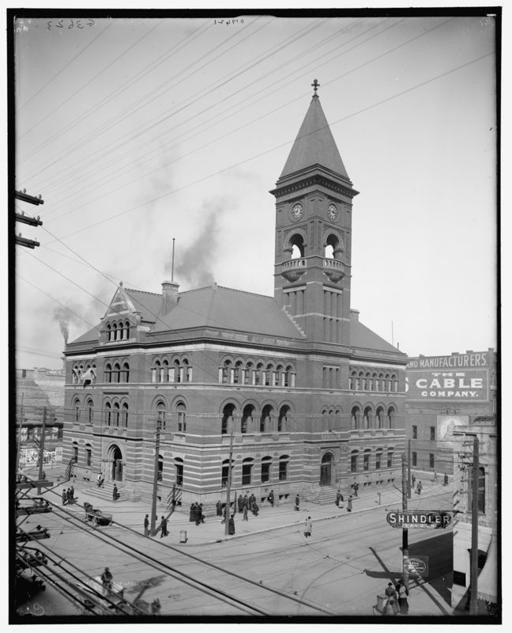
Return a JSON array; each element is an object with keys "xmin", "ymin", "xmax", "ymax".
[
  {"xmin": 335, "ymin": 481, "xmax": 360, "ymax": 512},
  {"xmin": 215, "ymin": 493, "xmax": 260, "ymax": 535},
  {"xmin": 143, "ymin": 513, "xmax": 150, "ymax": 536},
  {"xmin": 159, "ymin": 515, "xmax": 168, "ymax": 537},
  {"xmin": 189, "ymin": 501, "xmax": 205, "ymax": 526},
  {"xmin": 268, "ymin": 488, "xmax": 275, "ymax": 506},
  {"xmin": 112, "ymin": 483, "xmax": 118, "ymax": 500},
  {"xmin": 84, "ymin": 502, "xmax": 99, "ymax": 521},
  {"xmin": 100, "ymin": 566, "xmax": 113, "ymax": 594},
  {"xmin": 381, "ymin": 579, "xmax": 410, "ymax": 616},
  {"xmin": 416, "ymin": 480, "xmax": 423, "ymax": 494},
  {"xmin": 295, "ymin": 493, "xmax": 301, "ymax": 511},
  {"xmin": 303, "ymin": 515, "xmax": 313, "ymax": 540},
  {"xmin": 62, "ymin": 485, "xmax": 75, "ymax": 504},
  {"xmin": 443, "ymin": 472, "xmax": 449, "ymax": 485},
  {"xmin": 96, "ymin": 471, "xmax": 103, "ymax": 486}
]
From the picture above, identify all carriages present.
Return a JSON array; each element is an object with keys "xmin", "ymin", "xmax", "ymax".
[
  {"xmin": 80, "ymin": 576, "xmax": 161, "ymax": 615},
  {"xmin": 84, "ymin": 502, "xmax": 112, "ymax": 526}
]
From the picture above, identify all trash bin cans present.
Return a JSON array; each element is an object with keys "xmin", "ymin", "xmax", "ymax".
[{"xmin": 180, "ymin": 530, "xmax": 188, "ymax": 543}]
